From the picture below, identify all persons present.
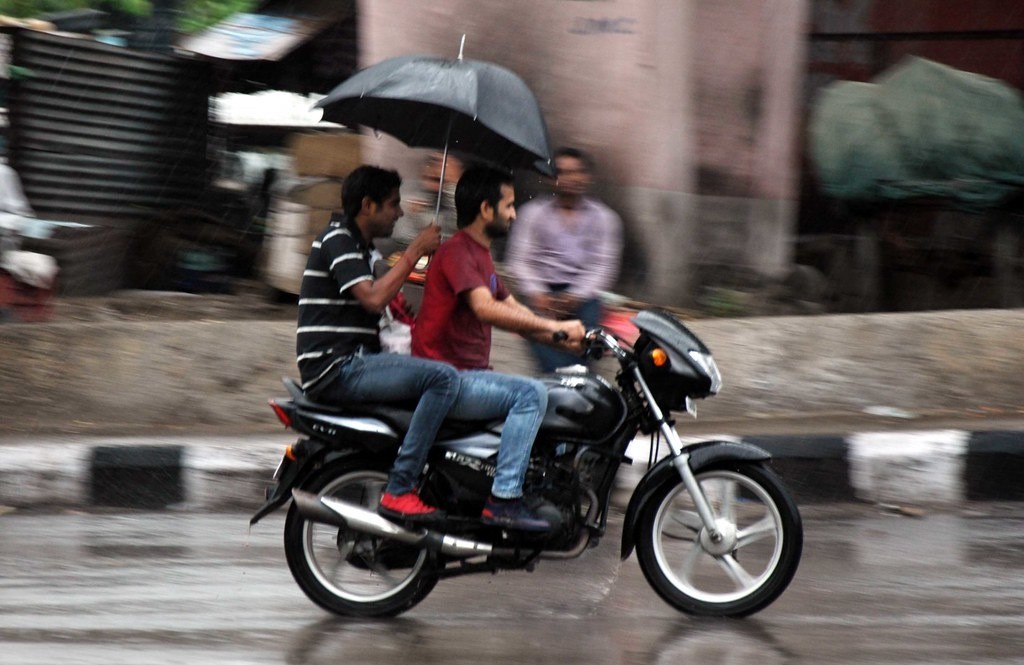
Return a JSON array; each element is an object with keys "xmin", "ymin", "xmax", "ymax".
[
  {"xmin": 509, "ymin": 142, "xmax": 626, "ymax": 371},
  {"xmin": 411, "ymin": 161, "xmax": 587, "ymax": 534},
  {"xmin": 296, "ymin": 163, "xmax": 463, "ymax": 524}
]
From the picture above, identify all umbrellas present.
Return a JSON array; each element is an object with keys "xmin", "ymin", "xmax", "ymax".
[{"xmin": 306, "ymin": 30, "xmax": 556, "ymax": 271}]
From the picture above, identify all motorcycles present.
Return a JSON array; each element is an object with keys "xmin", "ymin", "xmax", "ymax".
[{"xmin": 248, "ymin": 308, "xmax": 803, "ymax": 621}]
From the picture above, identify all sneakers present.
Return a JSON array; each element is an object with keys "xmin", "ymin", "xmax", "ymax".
[
  {"xmin": 481, "ymin": 497, "xmax": 550, "ymax": 530},
  {"xmin": 375, "ymin": 491, "xmax": 445, "ymax": 522}
]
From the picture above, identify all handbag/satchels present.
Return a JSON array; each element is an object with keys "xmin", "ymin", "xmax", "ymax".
[{"xmin": 378, "ymin": 304, "xmax": 412, "ymax": 356}]
